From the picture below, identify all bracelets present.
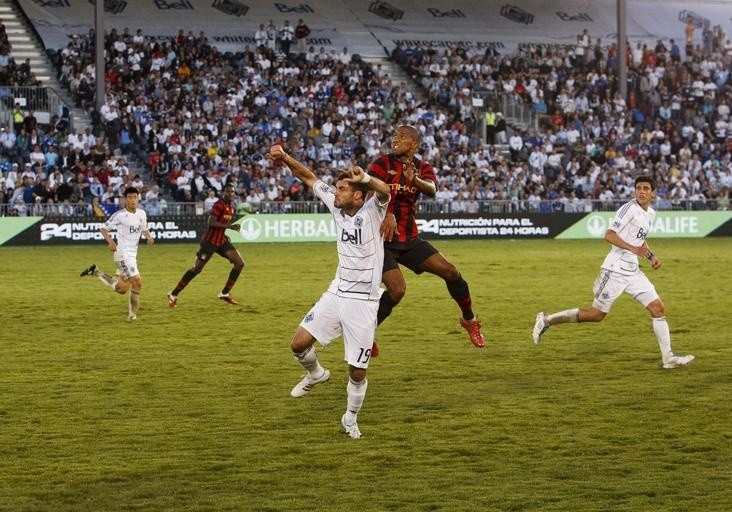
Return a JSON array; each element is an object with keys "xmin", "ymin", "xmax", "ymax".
[{"xmin": 647, "ymin": 252, "xmax": 653, "ymax": 260}]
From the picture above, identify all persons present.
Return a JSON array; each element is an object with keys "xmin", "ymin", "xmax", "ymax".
[
  {"xmin": 0, "ymin": 19, "xmax": 732, "ymax": 218},
  {"xmin": 167, "ymin": 184, "xmax": 245, "ymax": 309},
  {"xmin": 267, "ymin": 145, "xmax": 391, "ymax": 441},
  {"xmin": 80, "ymin": 186, "xmax": 154, "ymax": 319},
  {"xmin": 368, "ymin": 126, "xmax": 485, "ymax": 356},
  {"xmin": 532, "ymin": 176, "xmax": 696, "ymax": 370}
]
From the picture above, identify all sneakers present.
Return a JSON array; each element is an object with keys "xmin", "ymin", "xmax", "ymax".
[
  {"xmin": 460, "ymin": 317, "xmax": 486, "ymax": 347},
  {"xmin": 340, "ymin": 413, "xmax": 363, "ymax": 439},
  {"xmin": 291, "ymin": 369, "xmax": 330, "ymax": 398},
  {"xmin": 167, "ymin": 291, "xmax": 178, "ymax": 308},
  {"xmin": 80, "ymin": 264, "xmax": 97, "ymax": 277},
  {"xmin": 532, "ymin": 312, "xmax": 550, "ymax": 344},
  {"xmin": 663, "ymin": 354, "xmax": 695, "ymax": 369},
  {"xmin": 217, "ymin": 292, "xmax": 237, "ymax": 304},
  {"xmin": 370, "ymin": 341, "xmax": 379, "ymax": 358}
]
